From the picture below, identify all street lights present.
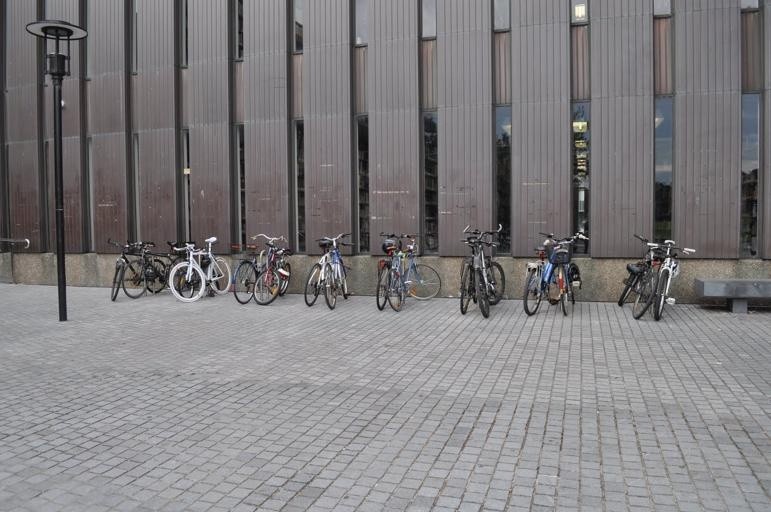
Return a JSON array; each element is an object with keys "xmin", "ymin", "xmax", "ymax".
[{"xmin": 23, "ymin": 15, "xmax": 89, "ymax": 321}]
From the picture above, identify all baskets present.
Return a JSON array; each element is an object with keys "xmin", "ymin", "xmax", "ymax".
[
  {"xmin": 231, "ymin": 249, "xmax": 256, "ymax": 262},
  {"xmin": 547, "ymin": 244, "xmax": 572, "ymax": 265}
]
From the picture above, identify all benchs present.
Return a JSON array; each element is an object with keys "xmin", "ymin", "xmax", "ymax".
[{"xmin": 696, "ymin": 277, "xmax": 770, "ymax": 314}]
